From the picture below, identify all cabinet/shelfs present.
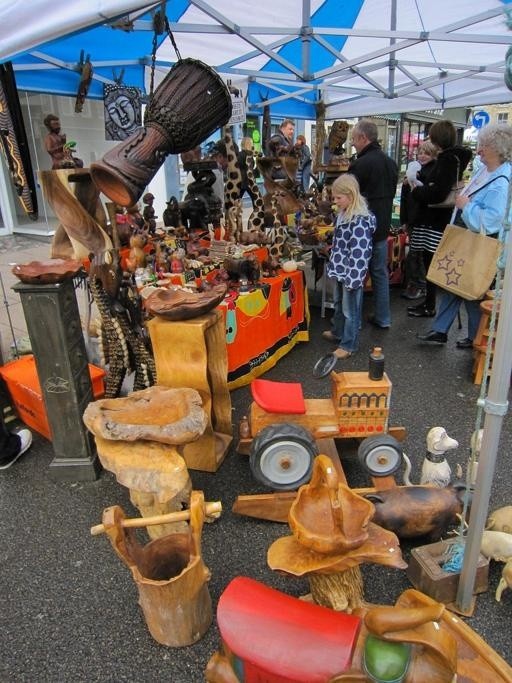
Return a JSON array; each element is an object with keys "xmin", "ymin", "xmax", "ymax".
[
  {"xmin": 84, "ymin": 224, "xmax": 312, "ymax": 393},
  {"xmin": 289, "ymin": 200, "xmax": 412, "ymax": 318}
]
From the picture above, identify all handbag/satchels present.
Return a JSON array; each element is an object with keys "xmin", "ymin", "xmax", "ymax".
[
  {"xmin": 428, "ymin": 180, "xmax": 466, "ymax": 208},
  {"xmin": 426, "ymin": 224, "xmax": 499, "ymax": 301}
]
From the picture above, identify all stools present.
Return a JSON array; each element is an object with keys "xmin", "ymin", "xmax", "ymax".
[{"xmin": 472, "ymin": 289, "xmax": 503, "ymax": 386}]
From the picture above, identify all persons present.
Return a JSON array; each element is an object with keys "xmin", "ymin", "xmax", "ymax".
[
  {"xmin": 103, "ymin": 84, "xmax": 142, "ymax": 140},
  {"xmin": 43, "ymin": 113, "xmax": 82, "ymax": 169},
  {"xmin": 321, "ymin": 172, "xmax": 377, "ymax": 361},
  {"xmin": 271, "ymin": 119, "xmax": 296, "ymax": 152},
  {"xmin": 399, "ymin": 140, "xmax": 438, "ymax": 299},
  {"xmin": 0, "ymin": 399, "xmax": 33, "ymax": 469},
  {"xmin": 408, "ymin": 121, "xmax": 472, "ymax": 317},
  {"xmin": 348, "ymin": 121, "xmax": 399, "ymax": 329},
  {"xmin": 296, "ymin": 135, "xmax": 312, "ymax": 193},
  {"xmin": 416, "ymin": 120, "xmax": 512, "ymax": 349}
]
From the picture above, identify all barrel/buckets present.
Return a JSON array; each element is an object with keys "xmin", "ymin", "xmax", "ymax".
[{"xmin": 89, "ymin": 492, "xmax": 222, "ymax": 648}]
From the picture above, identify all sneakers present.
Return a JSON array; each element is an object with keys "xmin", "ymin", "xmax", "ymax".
[
  {"xmin": 367, "ymin": 312, "xmax": 376, "ymax": 323},
  {"xmin": 322, "ymin": 330, "xmax": 336, "ymax": 342},
  {"xmin": 332, "ymin": 347, "xmax": 352, "ymax": 360},
  {"xmin": 0, "ymin": 429, "xmax": 33, "ymax": 470}
]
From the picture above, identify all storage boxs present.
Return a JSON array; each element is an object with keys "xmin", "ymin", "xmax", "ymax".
[{"xmin": 0, "ymin": 353, "xmax": 106, "ymax": 444}]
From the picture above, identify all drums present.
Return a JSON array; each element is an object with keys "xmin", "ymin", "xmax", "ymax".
[{"xmin": 91, "ymin": 57, "xmax": 232, "ymax": 208}]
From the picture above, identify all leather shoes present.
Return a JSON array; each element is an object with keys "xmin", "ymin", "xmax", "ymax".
[
  {"xmin": 456, "ymin": 338, "xmax": 473, "ymax": 348},
  {"xmin": 400, "ymin": 287, "xmax": 437, "ymax": 318},
  {"xmin": 416, "ymin": 329, "xmax": 448, "ymax": 343}
]
